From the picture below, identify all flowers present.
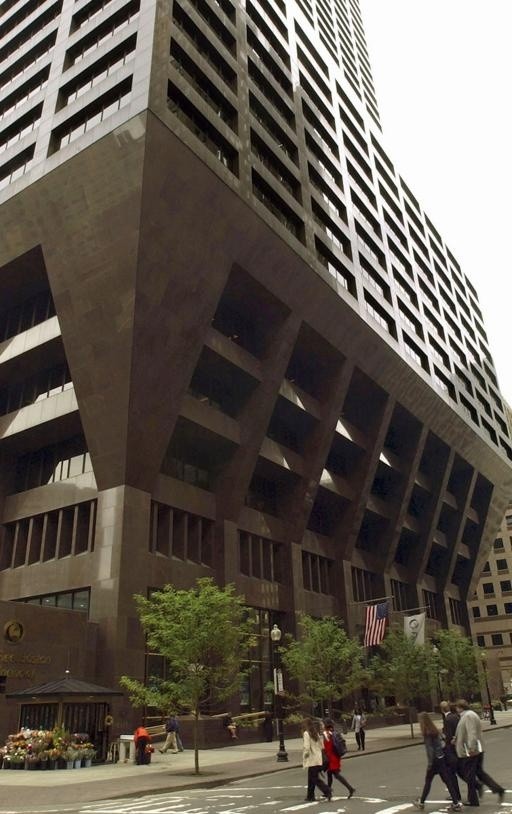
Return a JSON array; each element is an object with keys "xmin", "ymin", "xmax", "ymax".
[{"xmin": 0, "ymin": 727, "xmax": 97, "ymax": 762}]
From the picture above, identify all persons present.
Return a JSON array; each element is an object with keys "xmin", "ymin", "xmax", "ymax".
[
  {"xmin": 408, "ymin": 711, "xmax": 462, "ymax": 811},
  {"xmin": 450, "ymin": 698, "xmax": 506, "ymax": 808},
  {"xmin": 156, "ymin": 715, "xmax": 179, "ymax": 754},
  {"xmin": 350, "ymin": 704, "xmax": 368, "ymax": 751},
  {"xmin": 300, "ymin": 717, "xmax": 334, "ymax": 802},
  {"xmin": 133, "ymin": 720, "xmax": 152, "ymax": 765},
  {"xmin": 168, "ymin": 712, "xmax": 184, "ymax": 753},
  {"xmin": 263, "ymin": 710, "xmax": 274, "ymax": 742},
  {"xmin": 439, "ymin": 700, "xmax": 485, "ymax": 801},
  {"xmin": 222, "ymin": 710, "xmax": 239, "ymax": 740},
  {"xmin": 319, "ymin": 715, "xmax": 356, "ymax": 799}
]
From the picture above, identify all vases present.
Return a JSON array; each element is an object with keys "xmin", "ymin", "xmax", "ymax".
[{"xmin": 0, "ymin": 759, "xmax": 92, "ymax": 770}]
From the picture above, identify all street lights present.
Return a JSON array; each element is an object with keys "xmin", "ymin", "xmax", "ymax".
[
  {"xmin": 271, "ymin": 621, "xmax": 289, "ymax": 761},
  {"xmin": 480, "ymin": 652, "xmax": 497, "ymax": 725},
  {"xmin": 431, "ymin": 646, "xmax": 448, "ymax": 733}
]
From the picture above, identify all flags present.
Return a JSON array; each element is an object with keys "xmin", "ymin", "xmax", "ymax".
[
  {"xmin": 361, "ymin": 601, "xmax": 390, "ymax": 648},
  {"xmin": 402, "ymin": 611, "xmax": 428, "ymax": 650}
]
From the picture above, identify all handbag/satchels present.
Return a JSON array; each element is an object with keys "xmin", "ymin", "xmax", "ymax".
[
  {"xmin": 320, "ymin": 749, "xmax": 329, "ymax": 773},
  {"xmin": 442, "ymin": 742, "xmax": 459, "ymax": 766},
  {"xmin": 330, "ymin": 731, "xmax": 347, "ymax": 756}
]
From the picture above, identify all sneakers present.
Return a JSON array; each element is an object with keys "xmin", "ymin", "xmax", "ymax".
[
  {"xmin": 446, "ymin": 805, "xmax": 464, "ymax": 813},
  {"xmin": 412, "ymin": 798, "xmax": 424, "ymax": 809}
]
